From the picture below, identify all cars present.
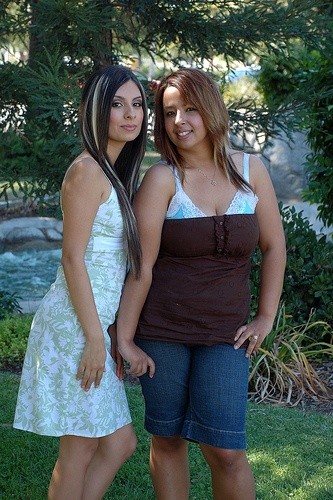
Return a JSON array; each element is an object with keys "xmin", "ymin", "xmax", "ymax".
[{"xmin": 220, "ymin": 65, "xmax": 261, "ymax": 83}]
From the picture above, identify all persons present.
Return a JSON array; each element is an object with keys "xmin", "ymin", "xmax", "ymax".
[
  {"xmin": 11, "ymin": 67, "xmax": 136, "ymax": 499},
  {"xmin": 116, "ymin": 68, "xmax": 288, "ymax": 499}
]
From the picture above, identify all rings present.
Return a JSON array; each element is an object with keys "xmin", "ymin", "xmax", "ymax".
[{"xmin": 253, "ymin": 335, "xmax": 258, "ymax": 339}]
